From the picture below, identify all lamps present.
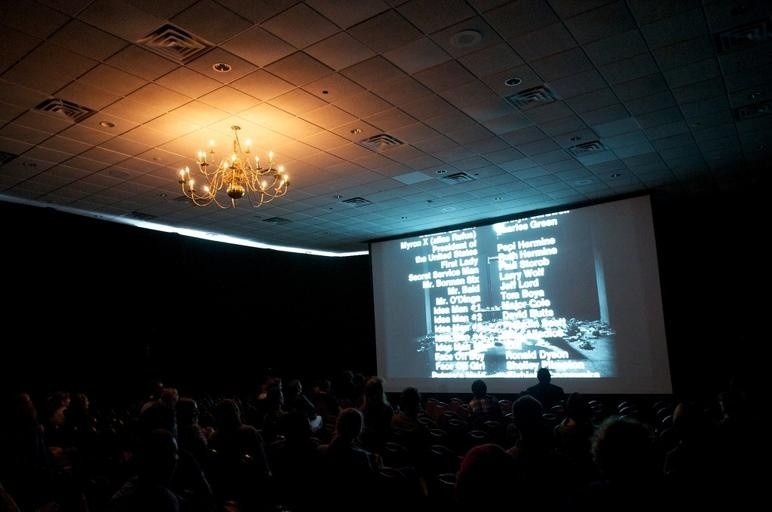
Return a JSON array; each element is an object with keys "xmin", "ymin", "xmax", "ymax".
[{"xmin": 178, "ymin": 126, "xmax": 288, "ymax": 209}]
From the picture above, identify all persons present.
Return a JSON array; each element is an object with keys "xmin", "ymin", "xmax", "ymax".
[{"xmin": 1, "ymin": 368, "xmax": 772, "ymax": 512}]
